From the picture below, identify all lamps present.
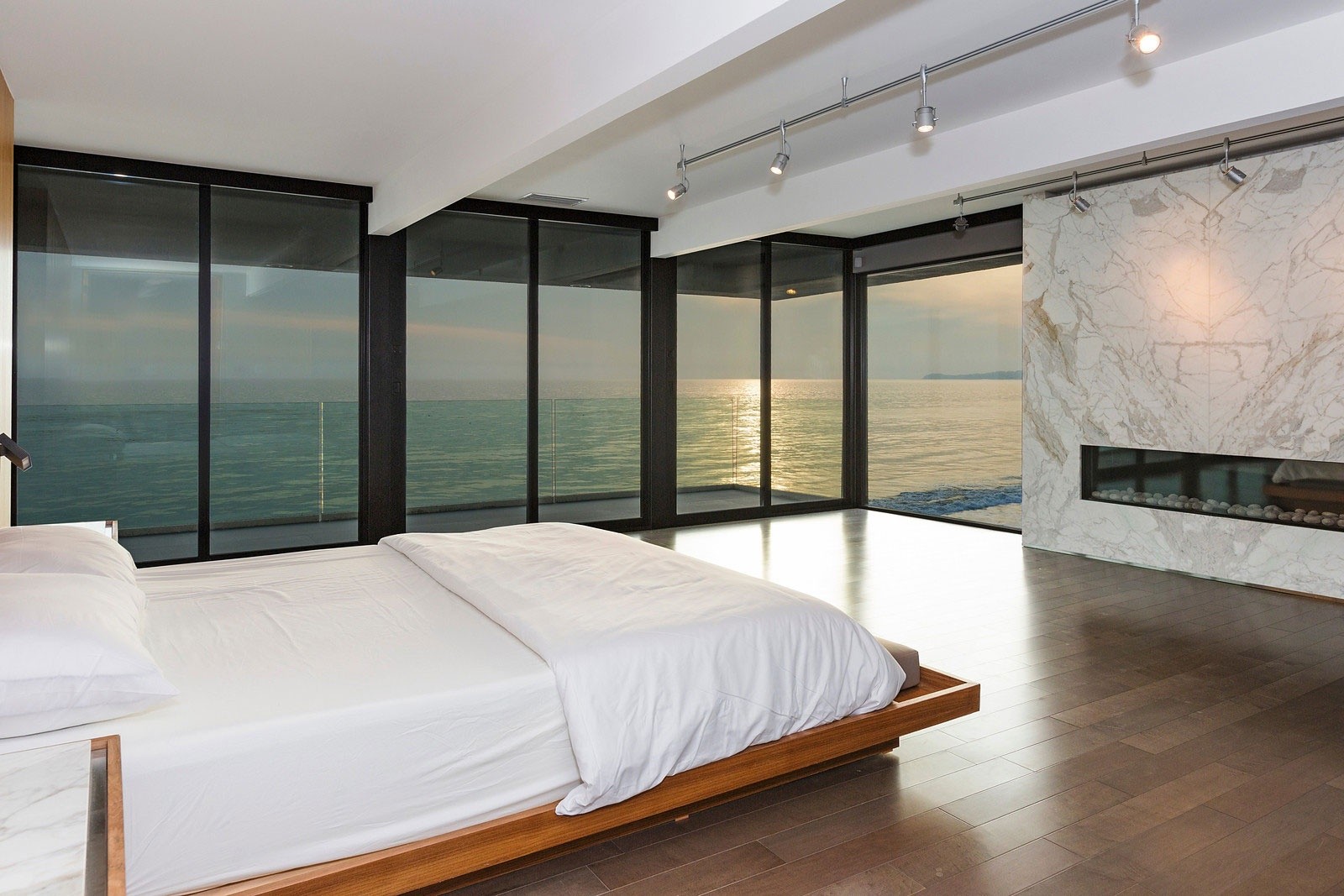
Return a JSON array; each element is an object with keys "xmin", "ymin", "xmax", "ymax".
[
  {"xmin": 954, "ymin": 196, "xmax": 968, "ymax": 234},
  {"xmin": 1125, "ymin": 0, "xmax": 1161, "ymax": 54},
  {"xmin": 770, "ymin": 119, "xmax": 792, "ymax": 175},
  {"xmin": 0, "ymin": 433, "xmax": 33, "ymax": 472},
  {"xmin": 667, "ymin": 160, "xmax": 690, "ymax": 201},
  {"xmin": 1068, "ymin": 170, "xmax": 1090, "ymax": 213},
  {"xmin": 1219, "ymin": 137, "xmax": 1246, "ymax": 184},
  {"xmin": 910, "ymin": 64, "xmax": 939, "ymax": 132}
]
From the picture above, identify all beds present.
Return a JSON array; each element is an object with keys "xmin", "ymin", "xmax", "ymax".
[{"xmin": 0, "ymin": 520, "xmax": 988, "ymax": 896}]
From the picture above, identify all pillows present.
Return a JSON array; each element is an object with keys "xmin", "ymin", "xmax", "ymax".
[{"xmin": 0, "ymin": 520, "xmax": 179, "ymax": 744}]
